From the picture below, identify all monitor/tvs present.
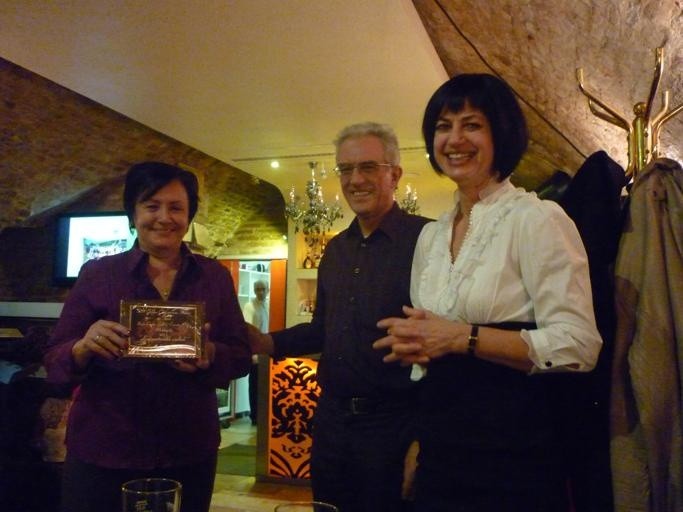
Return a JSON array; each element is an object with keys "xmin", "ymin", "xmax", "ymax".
[{"xmin": 52, "ymin": 210, "xmax": 139, "ymax": 289}]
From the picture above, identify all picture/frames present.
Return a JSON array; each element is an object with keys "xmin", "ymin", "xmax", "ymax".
[{"xmin": 119, "ymin": 299, "xmax": 206, "ymax": 360}]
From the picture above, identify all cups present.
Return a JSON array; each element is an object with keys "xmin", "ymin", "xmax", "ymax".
[
  {"xmin": 254, "ymin": 264, "xmax": 265, "ymax": 273},
  {"xmin": 116, "ymin": 477, "xmax": 182, "ymax": 512}
]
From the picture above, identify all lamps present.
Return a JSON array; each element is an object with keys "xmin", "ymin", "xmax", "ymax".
[
  {"xmin": 395, "ymin": 184, "xmax": 421, "ymax": 216},
  {"xmin": 284, "ymin": 160, "xmax": 344, "ymax": 250}
]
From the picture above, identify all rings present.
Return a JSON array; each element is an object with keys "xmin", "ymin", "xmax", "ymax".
[{"xmin": 93, "ymin": 333, "xmax": 102, "ymax": 343}]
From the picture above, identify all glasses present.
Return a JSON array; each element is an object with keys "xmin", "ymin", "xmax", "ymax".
[{"xmin": 335, "ymin": 161, "xmax": 396, "ymax": 174}]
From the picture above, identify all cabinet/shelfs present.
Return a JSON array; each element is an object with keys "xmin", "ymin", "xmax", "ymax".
[{"xmin": 234, "ymin": 269, "xmax": 270, "ymax": 413}]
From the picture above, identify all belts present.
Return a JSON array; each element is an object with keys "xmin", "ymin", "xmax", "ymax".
[{"xmin": 318, "ymin": 389, "xmax": 406, "ymax": 413}]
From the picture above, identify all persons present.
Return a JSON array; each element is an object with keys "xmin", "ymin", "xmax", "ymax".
[
  {"xmin": 242, "ymin": 279, "xmax": 271, "ymax": 426},
  {"xmin": 40, "ymin": 161, "xmax": 253, "ymax": 512},
  {"xmin": 369, "ymin": 71, "xmax": 604, "ymax": 511},
  {"xmin": 243, "ymin": 120, "xmax": 439, "ymax": 512}
]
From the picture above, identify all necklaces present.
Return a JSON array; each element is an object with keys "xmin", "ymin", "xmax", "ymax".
[{"xmin": 159, "ymin": 287, "xmax": 169, "ymax": 296}]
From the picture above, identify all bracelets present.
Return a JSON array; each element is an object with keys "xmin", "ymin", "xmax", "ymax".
[{"xmin": 466, "ymin": 323, "xmax": 480, "ymax": 360}]
